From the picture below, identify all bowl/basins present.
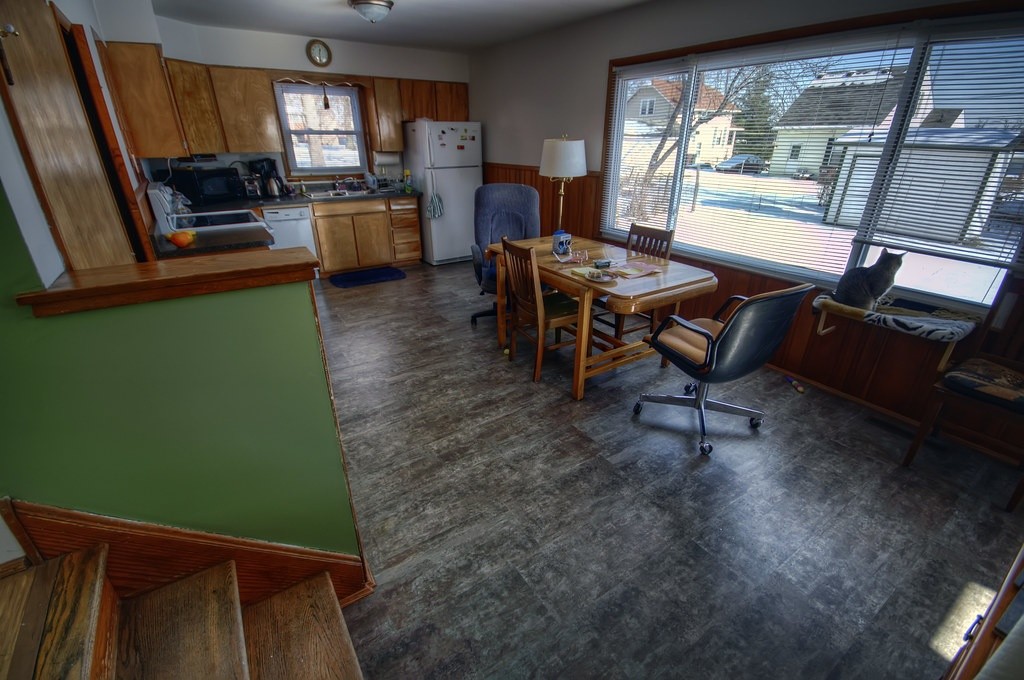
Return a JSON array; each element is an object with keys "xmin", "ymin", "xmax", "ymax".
[{"xmin": 568, "ymin": 250, "xmax": 588, "ymax": 264}]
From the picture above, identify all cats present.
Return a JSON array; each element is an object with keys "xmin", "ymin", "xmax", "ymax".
[{"xmin": 832, "ymin": 246, "xmax": 909, "ymax": 312}]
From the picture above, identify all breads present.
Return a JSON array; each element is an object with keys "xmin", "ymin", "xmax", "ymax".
[{"xmin": 588, "ymin": 270, "xmax": 602, "ymax": 278}]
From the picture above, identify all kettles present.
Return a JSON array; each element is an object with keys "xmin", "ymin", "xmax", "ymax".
[{"xmin": 267, "ymin": 176, "xmax": 284, "ymax": 197}]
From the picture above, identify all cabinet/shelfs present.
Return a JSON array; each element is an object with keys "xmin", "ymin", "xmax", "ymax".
[
  {"xmin": 166, "ymin": 58, "xmax": 284, "ymax": 157},
  {"xmin": 365, "ymin": 76, "xmax": 469, "ymax": 153},
  {"xmin": 105, "ymin": 41, "xmax": 192, "ymax": 160},
  {"xmin": 308, "ymin": 196, "xmax": 423, "ymax": 279}
]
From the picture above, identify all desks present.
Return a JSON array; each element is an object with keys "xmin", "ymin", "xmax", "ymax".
[{"xmin": 488, "ymin": 234, "xmax": 717, "ymax": 400}]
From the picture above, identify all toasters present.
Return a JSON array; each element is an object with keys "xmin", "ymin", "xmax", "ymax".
[{"xmin": 242, "ymin": 175, "xmax": 265, "ymax": 197}]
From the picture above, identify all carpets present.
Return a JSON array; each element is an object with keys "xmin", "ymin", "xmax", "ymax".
[{"xmin": 328, "ymin": 266, "xmax": 406, "ymax": 289}]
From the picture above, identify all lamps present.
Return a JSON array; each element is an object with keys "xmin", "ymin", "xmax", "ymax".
[
  {"xmin": 347, "ymin": 0, "xmax": 395, "ymax": 24},
  {"xmin": 539, "ymin": 136, "xmax": 588, "ymax": 252}
]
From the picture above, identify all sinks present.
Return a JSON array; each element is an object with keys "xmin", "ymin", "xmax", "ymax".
[{"xmin": 301, "ymin": 189, "xmax": 365, "ymax": 199}]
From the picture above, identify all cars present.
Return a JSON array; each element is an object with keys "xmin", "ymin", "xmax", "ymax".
[
  {"xmin": 990, "ymin": 192, "xmax": 1024, "ymax": 220},
  {"xmin": 716, "ymin": 153, "xmax": 763, "ymax": 175}
]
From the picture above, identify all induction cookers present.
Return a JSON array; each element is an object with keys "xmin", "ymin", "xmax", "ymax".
[{"xmin": 146, "ymin": 181, "xmax": 265, "ymax": 234}]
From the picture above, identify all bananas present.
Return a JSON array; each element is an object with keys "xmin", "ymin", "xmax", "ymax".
[{"xmin": 165, "ymin": 231, "xmax": 196, "ymax": 239}]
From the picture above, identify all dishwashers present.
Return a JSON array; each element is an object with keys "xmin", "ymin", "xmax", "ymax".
[{"xmin": 263, "ymin": 207, "xmax": 319, "ymax": 280}]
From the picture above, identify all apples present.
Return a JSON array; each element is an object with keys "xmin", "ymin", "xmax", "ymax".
[{"xmin": 170, "ymin": 232, "xmax": 193, "ymax": 247}]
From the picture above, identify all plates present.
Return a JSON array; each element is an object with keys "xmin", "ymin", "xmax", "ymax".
[{"xmin": 584, "ymin": 273, "xmax": 615, "ymax": 282}]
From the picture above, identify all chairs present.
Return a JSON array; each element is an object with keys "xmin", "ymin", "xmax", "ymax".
[
  {"xmin": 500, "ymin": 236, "xmax": 595, "ymax": 383},
  {"xmin": 635, "ymin": 283, "xmax": 815, "ymax": 455},
  {"xmin": 902, "ymin": 274, "xmax": 1024, "ymax": 512},
  {"xmin": 592, "ymin": 223, "xmax": 675, "ymax": 360},
  {"xmin": 471, "ymin": 183, "xmax": 540, "ymax": 326}
]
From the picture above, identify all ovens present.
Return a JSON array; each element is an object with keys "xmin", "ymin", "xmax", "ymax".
[{"xmin": 154, "ymin": 166, "xmax": 242, "ymax": 203}]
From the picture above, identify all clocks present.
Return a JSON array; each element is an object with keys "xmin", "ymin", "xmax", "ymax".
[{"xmin": 305, "ymin": 37, "xmax": 334, "ymax": 66}]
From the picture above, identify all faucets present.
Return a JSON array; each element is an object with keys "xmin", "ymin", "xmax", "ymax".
[{"xmin": 335, "ymin": 176, "xmax": 339, "ymax": 190}]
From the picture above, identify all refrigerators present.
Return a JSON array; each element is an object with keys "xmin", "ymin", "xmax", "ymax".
[{"xmin": 405, "ymin": 117, "xmax": 483, "ymax": 267}]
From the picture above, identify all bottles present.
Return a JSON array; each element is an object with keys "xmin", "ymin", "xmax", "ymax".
[
  {"xmin": 404, "ymin": 170, "xmax": 412, "ymax": 185},
  {"xmin": 300, "ymin": 180, "xmax": 306, "ymax": 192}
]
